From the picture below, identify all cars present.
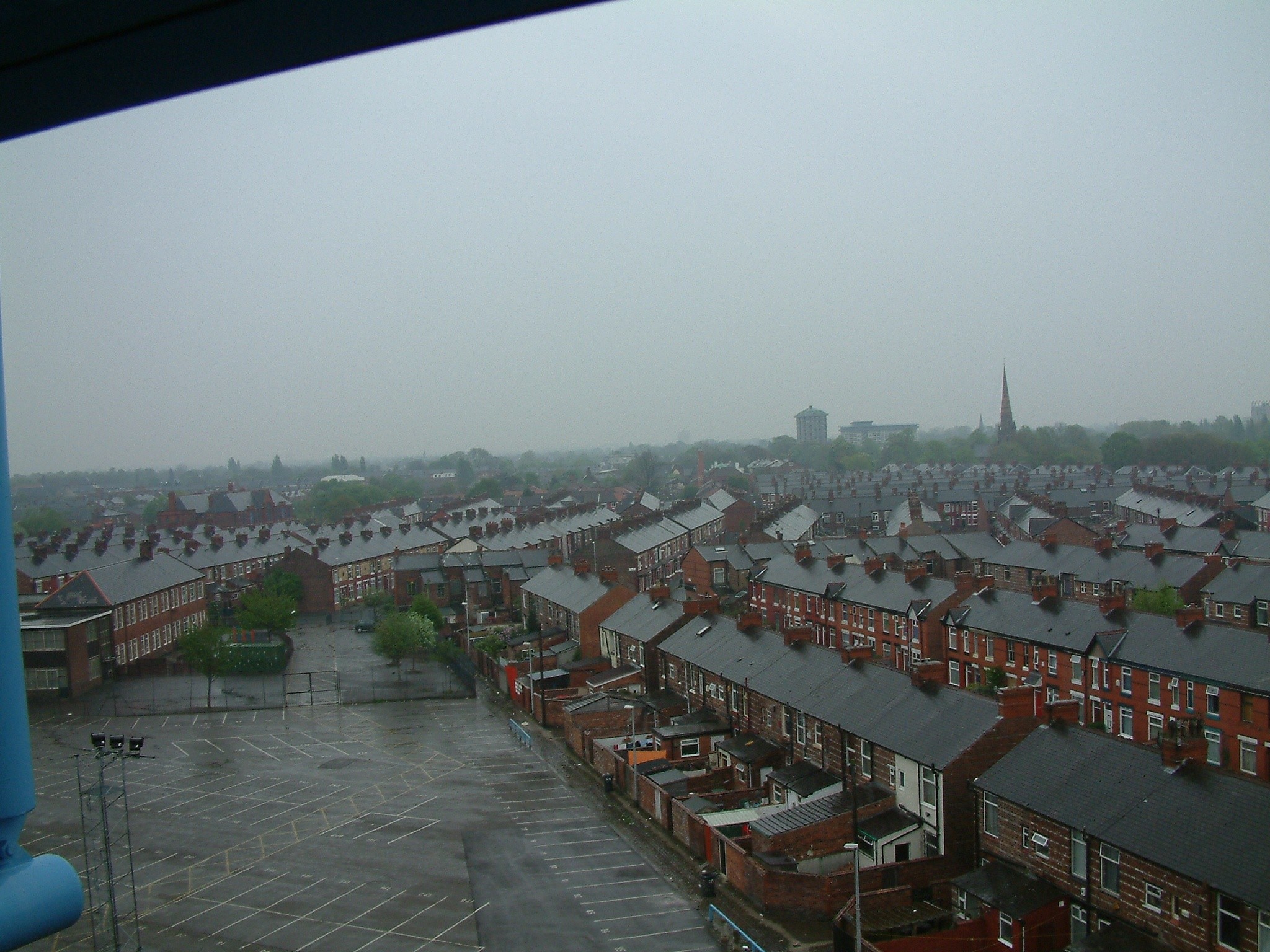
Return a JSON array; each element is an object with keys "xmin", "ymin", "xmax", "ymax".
[{"xmin": 354, "ymin": 620, "xmax": 379, "ymax": 633}]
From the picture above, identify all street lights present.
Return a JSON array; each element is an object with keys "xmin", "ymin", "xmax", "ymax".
[
  {"xmin": 624, "ymin": 705, "xmax": 638, "ymax": 805},
  {"xmin": 844, "ymin": 843, "xmax": 861, "ymax": 952},
  {"xmin": 523, "ymin": 642, "xmax": 534, "ymax": 714},
  {"xmin": 462, "ymin": 603, "xmax": 470, "ymax": 657}
]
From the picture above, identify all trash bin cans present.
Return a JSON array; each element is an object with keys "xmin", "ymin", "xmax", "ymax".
[
  {"xmin": 700, "ymin": 871, "xmax": 717, "ymax": 898},
  {"xmin": 602, "ymin": 773, "xmax": 615, "ymax": 792}
]
